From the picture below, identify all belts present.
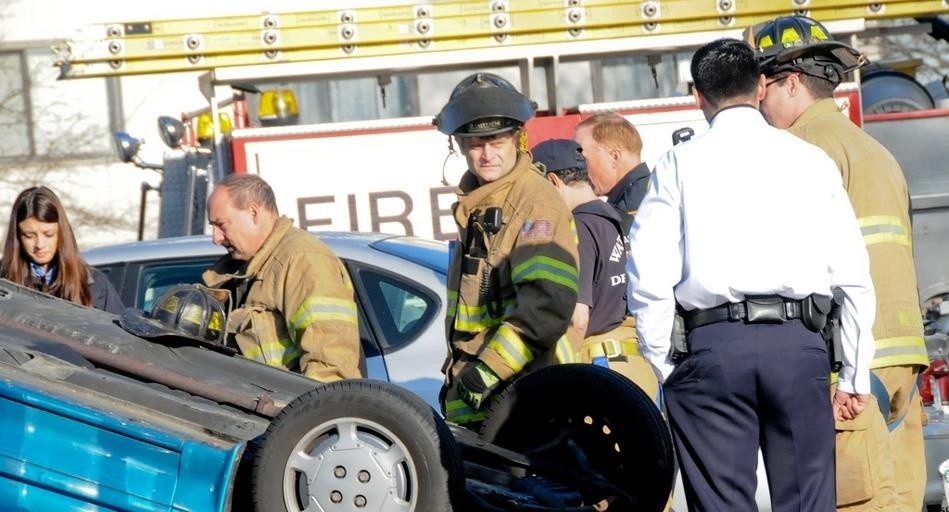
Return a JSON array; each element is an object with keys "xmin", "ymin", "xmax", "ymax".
[{"xmin": 685, "ymin": 296, "xmax": 805, "ymax": 331}]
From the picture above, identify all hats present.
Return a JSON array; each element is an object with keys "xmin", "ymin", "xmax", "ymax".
[{"xmin": 529, "ymin": 138, "xmax": 588, "ymax": 174}]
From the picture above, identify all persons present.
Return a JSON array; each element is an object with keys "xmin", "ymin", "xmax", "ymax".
[
  {"xmin": 740, "ymin": 14, "xmax": 931, "ymax": 512},
  {"xmin": 0, "ymin": 183, "xmax": 125, "ymax": 315},
  {"xmin": 200, "ymin": 173, "xmax": 369, "ymax": 383},
  {"xmin": 623, "ymin": 37, "xmax": 879, "ymax": 512},
  {"xmin": 572, "ymin": 108, "xmax": 651, "ymax": 236},
  {"xmin": 430, "ymin": 71, "xmax": 582, "ymax": 432},
  {"xmin": 530, "ymin": 137, "xmax": 660, "ymax": 404}
]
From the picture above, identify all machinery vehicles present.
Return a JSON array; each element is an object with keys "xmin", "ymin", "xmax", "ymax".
[{"xmin": 53, "ymin": 1, "xmax": 948, "ymax": 365}]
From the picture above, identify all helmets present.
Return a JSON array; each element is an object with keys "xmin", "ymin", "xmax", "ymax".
[
  {"xmin": 117, "ymin": 283, "xmax": 239, "ymax": 353},
  {"xmin": 742, "ymin": 15, "xmax": 871, "ymax": 85},
  {"xmin": 430, "ymin": 72, "xmax": 538, "ymax": 138}
]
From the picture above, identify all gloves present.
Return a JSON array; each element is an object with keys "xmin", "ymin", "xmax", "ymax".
[{"xmin": 456, "ymin": 361, "xmax": 501, "ymax": 414}]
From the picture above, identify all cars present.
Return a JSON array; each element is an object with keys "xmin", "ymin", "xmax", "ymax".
[
  {"xmin": 1, "ymin": 277, "xmax": 675, "ymax": 511},
  {"xmin": 914, "ymin": 311, "xmax": 949, "ymax": 505},
  {"xmin": 77, "ymin": 230, "xmax": 772, "ymax": 511}
]
